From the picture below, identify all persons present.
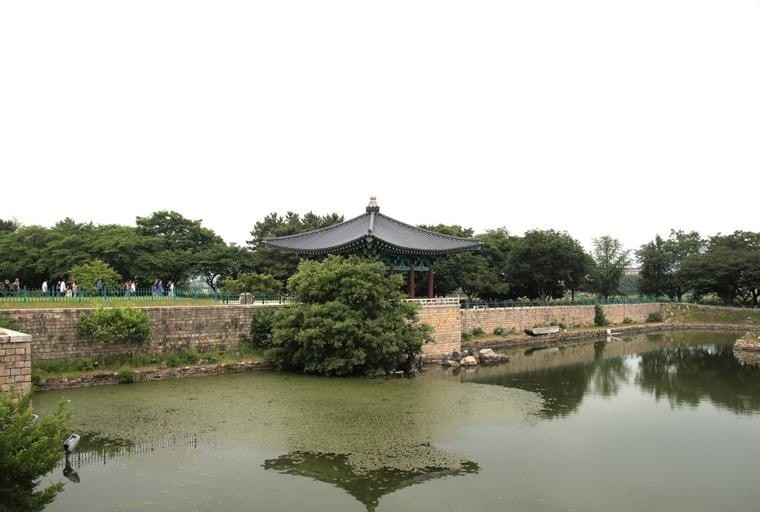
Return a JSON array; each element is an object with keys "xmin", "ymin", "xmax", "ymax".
[{"xmin": 3, "ymin": 276, "xmax": 175, "ymax": 300}]
